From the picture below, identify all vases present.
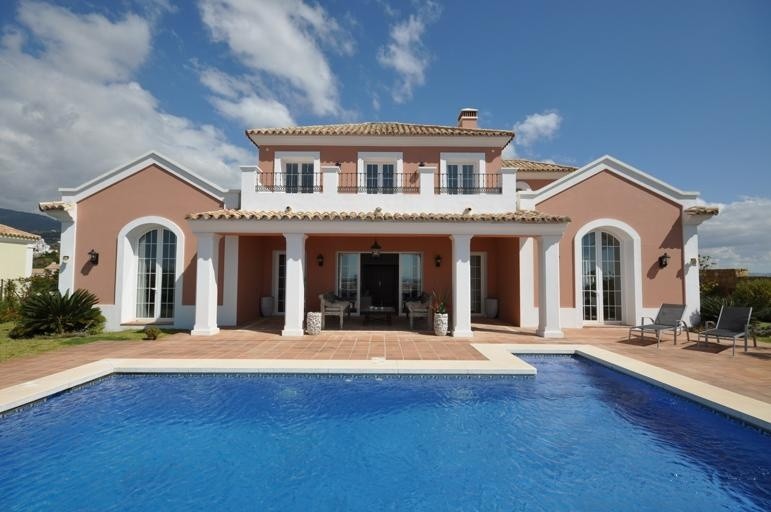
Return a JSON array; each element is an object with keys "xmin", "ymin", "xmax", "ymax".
[
  {"xmin": 307, "ymin": 310, "xmax": 323, "ymax": 336},
  {"xmin": 486, "ymin": 297, "xmax": 500, "ymax": 320},
  {"xmin": 260, "ymin": 295, "xmax": 275, "ymax": 317}
]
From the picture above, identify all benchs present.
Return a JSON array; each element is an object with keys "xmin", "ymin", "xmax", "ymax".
[
  {"xmin": 317, "ymin": 291, "xmax": 353, "ymax": 329},
  {"xmin": 404, "ymin": 291, "xmax": 435, "ymax": 329}
]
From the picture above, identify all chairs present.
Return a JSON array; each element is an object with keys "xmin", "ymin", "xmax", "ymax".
[
  {"xmin": 625, "ymin": 303, "xmax": 693, "ymax": 348},
  {"xmin": 692, "ymin": 302, "xmax": 761, "ymax": 355}
]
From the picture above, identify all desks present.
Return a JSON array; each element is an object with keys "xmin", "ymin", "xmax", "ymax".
[{"xmin": 361, "ymin": 305, "xmax": 397, "ymax": 327}]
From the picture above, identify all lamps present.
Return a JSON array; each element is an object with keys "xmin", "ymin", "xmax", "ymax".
[
  {"xmin": 435, "ymin": 255, "xmax": 442, "ymax": 267},
  {"xmin": 661, "ymin": 254, "xmax": 671, "ymax": 269},
  {"xmin": 317, "ymin": 253, "xmax": 324, "ymax": 266},
  {"xmin": 372, "ymin": 239, "xmax": 382, "ymax": 258},
  {"xmin": 87, "ymin": 249, "xmax": 99, "ymax": 265}
]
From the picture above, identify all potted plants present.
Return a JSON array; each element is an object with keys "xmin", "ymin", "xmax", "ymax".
[{"xmin": 429, "ymin": 287, "xmax": 451, "ymax": 336}]
